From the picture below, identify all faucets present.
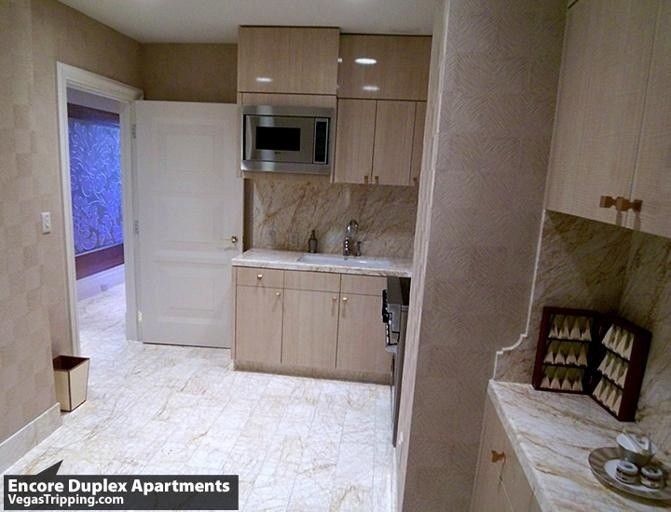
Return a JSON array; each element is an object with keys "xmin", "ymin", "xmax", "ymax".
[{"xmin": 343, "ymin": 220, "xmax": 359, "ymax": 256}]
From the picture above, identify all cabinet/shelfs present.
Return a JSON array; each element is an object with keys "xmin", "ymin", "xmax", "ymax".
[
  {"xmin": 542, "ymin": 0, "xmax": 671, "ymax": 244},
  {"xmin": 231, "ymin": 266, "xmax": 284, "ymax": 365},
  {"xmin": 471, "ymin": 393, "xmax": 542, "ymax": 512},
  {"xmin": 331, "ymin": 99, "xmax": 416, "ymax": 189},
  {"xmin": 409, "ymin": 101, "xmax": 427, "ymax": 188},
  {"xmin": 281, "ymin": 270, "xmax": 394, "ymax": 373}
]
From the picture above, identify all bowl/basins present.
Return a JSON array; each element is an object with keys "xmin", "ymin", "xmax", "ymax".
[{"xmin": 614, "ymin": 432, "xmax": 659, "ymax": 462}]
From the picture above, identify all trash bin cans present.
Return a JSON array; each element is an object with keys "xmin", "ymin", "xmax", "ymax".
[{"xmin": 52, "ymin": 355, "xmax": 89, "ymax": 412}]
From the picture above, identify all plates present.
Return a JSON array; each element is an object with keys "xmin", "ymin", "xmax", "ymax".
[{"xmin": 588, "ymin": 440, "xmax": 670, "ymax": 502}]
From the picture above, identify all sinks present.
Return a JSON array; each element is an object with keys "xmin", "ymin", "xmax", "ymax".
[{"xmin": 297, "ymin": 253, "xmax": 392, "ymax": 268}]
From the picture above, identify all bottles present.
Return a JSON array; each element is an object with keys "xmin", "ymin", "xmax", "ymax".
[{"xmin": 308, "ymin": 230, "xmax": 318, "ymax": 253}]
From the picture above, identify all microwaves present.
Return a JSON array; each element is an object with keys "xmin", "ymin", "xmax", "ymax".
[{"xmin": 239, "ymin": 104, "xmax": 335, "ymax": 175}]
